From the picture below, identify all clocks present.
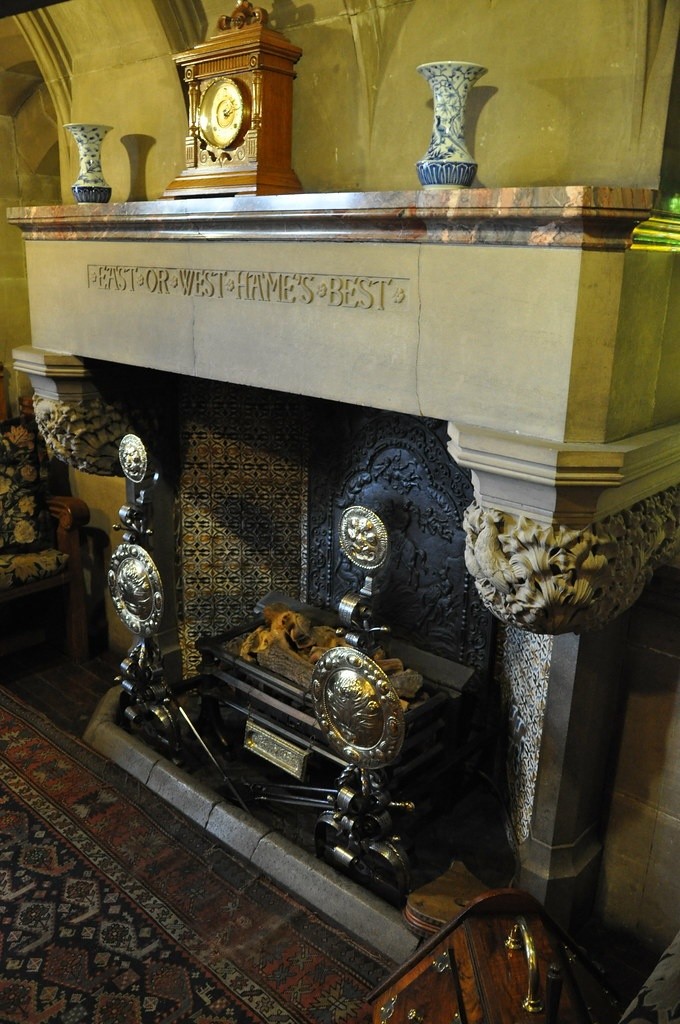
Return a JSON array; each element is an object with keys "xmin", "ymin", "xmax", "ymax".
[{"xmin": 156, "ymin": 0, "xmax": 302, "ymax": 200}]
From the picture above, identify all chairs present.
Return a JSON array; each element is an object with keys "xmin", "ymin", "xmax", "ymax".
[{"xmin": 0, "ymin": 361, "xmax": 90, "ymax": 666}]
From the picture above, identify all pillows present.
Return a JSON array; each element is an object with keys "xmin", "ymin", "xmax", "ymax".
[{"xmin": 0, "ymin": 417, "xmax": 54, "ymax": 556}]
